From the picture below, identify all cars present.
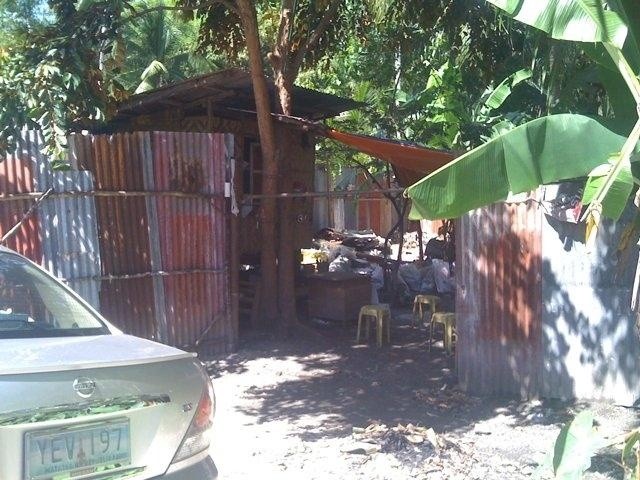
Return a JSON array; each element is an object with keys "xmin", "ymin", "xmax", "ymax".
[{"xmin": 0, "ymin": 243, "xmax": 219, "ymax": 480}]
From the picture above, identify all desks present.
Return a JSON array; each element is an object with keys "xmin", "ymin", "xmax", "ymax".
[{"xmin": 308, "ymin": 273, "xmax": 374, "ymax": 329}]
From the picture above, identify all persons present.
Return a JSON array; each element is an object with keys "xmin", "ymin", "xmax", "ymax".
[{"xmin": 423, "ymin": 223, "xmax": 456, "ymax": 264}]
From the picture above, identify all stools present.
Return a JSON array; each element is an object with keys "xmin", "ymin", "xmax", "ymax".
[
  {"xmin": 357, "ymin": 305, "xmax": 391, "ymax": 348},
  {"xmin": 410, "ymin": 294, "xmax": 440, "ymax": 330},
  {"xmin": 428, "ymin": 313, "xmax": 457, "ymax": 354}
]
